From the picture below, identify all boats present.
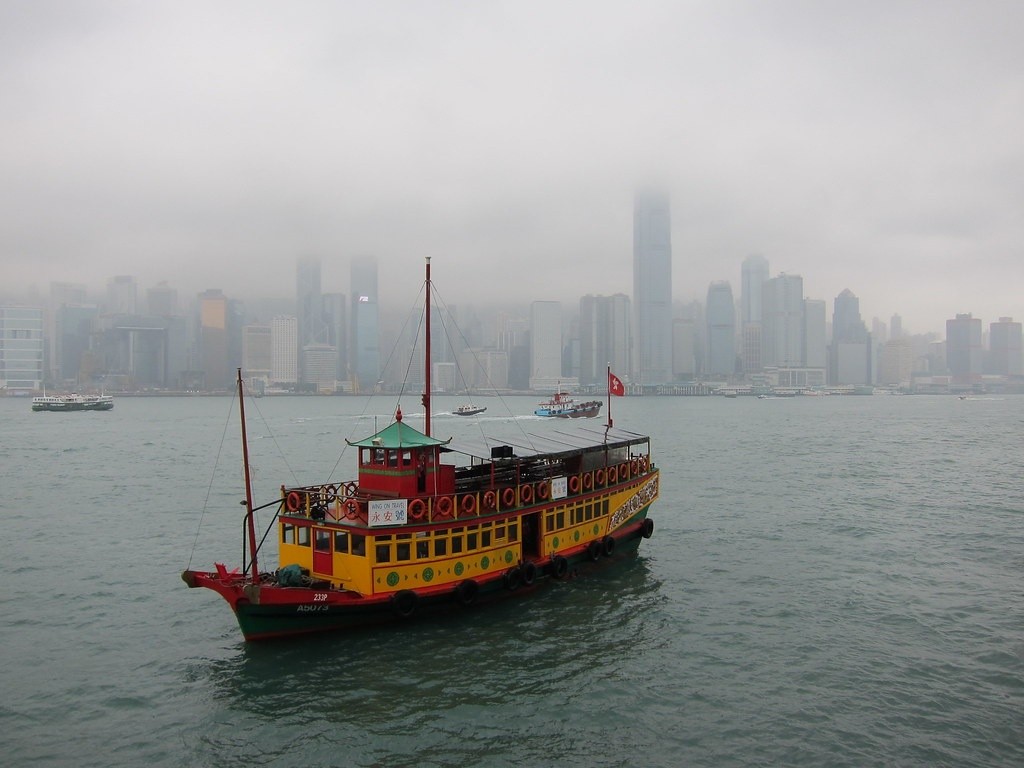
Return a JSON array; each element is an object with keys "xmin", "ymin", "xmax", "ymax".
[
  {"xmin": 758, "ymin": 395, "xmax": 765, "ymax": 398},
  {"xmin": 725, "ymin": 391, "xmax": 737, "ymax": 398},
  {"xmin": 181, "ymin": 257, "xmax": 658, "ymax": 643},
  {"xmin": 534, "ymin": 381, "xmax": 603, "ymax": 418},
  {"xmin": 32, "ymin": 384, "xmax": 113, "ymax": 412},
  {"xmin": 451, "ymin": 404, "xmax": 487, "ymax": 416}
]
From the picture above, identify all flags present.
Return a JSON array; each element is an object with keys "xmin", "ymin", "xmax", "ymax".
[{"xmin": 609, "ymin": 373, "xmax": 624, "ymax": 396}]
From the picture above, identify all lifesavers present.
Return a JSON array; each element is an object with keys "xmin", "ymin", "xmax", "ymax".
[
  {"xmin": 436, "ymin": 497, "xmax": 452, "ymax": 516},
  {"xmin": 389, "ymin": 589, "xmax": 418, "ymax": 617},
  {"xmin": 588, "ymin": 540, "xmax": 602, "ymax": 561},
  {"xmin": 538, "ymin": 481, "xmax": 550, "ymax": 499},
  {"xmin": 630, "ymin": 461, "xmax": 638, "ymax": 475},
  {"xmin": 483, "ymin": 491, "xmax": 496, "ymax": 509},
  {"xmin": 583, "ymin": 472, "xmax": 592, "ymax": 489},
  {"xmin": 601, "ymin": 535, "xmax": 615, "ymax": 557},
  {"xmin": 342, "ymin": 500, "xmax": 360, "ymax": 519},
  {"xmin": 620, "ymin": 464, "xmax": 627, "ymax": 478},
  {"xmin": 325, "ymin": 485, "xmax": 337, "ymax": 502},
  {"xmin": 287, "ymin": 492, "xmax": 300, "ymax": 512},
  {"xmin": 408, "ymin": 499, "xmax": 426, "ymax": 519},
  {"xmin": 505, "ymin": 567, "xmax": 521, "ymax": 591},
  {"xmin": 551, "ymin": 556, "xmax": 567, "ymax": 577},
  {"xmin": 521, "ymin": 485, "xmax": 532, "ymax": 502},
  {"xmin": 345, "ymin": 482, "xmax": 358, "ymax": 500},
  {"xmin": 457, "ymin": 579, "xmax": 478, "ymax": 601},
  {"xmin": 596, "ymin": 470, "xmax": 604, "ymax": 485},
  {"xmin": 641, "ymin": 517, "xmax": 654, "ymax": 539},
  {"xmin": 570, "ymin": 477, "xmax": 580, "ymax": 492},
  {"xmin": 608, "ymin": 467, "xmax": 616, "ymax": 482},
  {"xmin": 520, "ymin": 561, "xmax": 537, "ymax": 586},
  {"xmin": 462, "ymin": 494, "xmax": 476, "ymax": 513},
  {"xmin": 503, "ymin": 488, "xmax": 515, "ymax": 506},
  {"xmin": 640, "ymin": 458, "xmax": 648, "ymax": 471}
]
